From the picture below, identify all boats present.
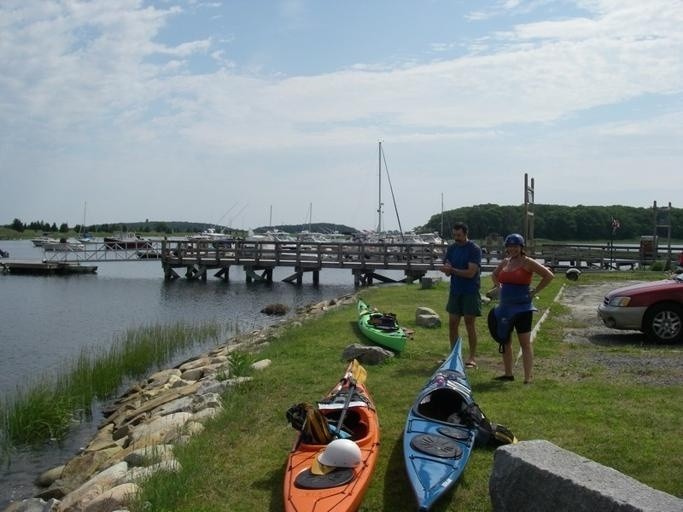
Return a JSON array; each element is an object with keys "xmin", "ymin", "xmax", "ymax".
[
  {"xmin": 138, "ymin": 250, "xmax": 162, "ymax": 258},
  {"xmin": 1, "ymin": 260, "xmax": 98, "ymax": 275},
  {"xmin": 356, "ymin": 297, "xmax": 409, "ymax": 353},
  {"xmin": 282, "ymin": 361, "xmax": 380, "ymax": 512},
  {"xmin": 188, "ymin": 228, "xmax": 233, "ymax": 248},
  {"xmin": 103, "ymin": 231, "xmax": 152, "ymax": 248},
  {"xmin": 32, "ymin": 235, "xmax": 80, "ymax": 246},
  {"xmin": 402, "ymin": 338, "xmax": 480, "ymax": 508}
]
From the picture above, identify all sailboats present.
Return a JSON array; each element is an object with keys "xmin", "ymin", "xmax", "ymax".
[{"xmin": 241, "ymin": 140, "xmax": 447, "ymax": 261}]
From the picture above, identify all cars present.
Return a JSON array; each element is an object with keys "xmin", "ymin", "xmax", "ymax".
[{"xmin": 597, "ymin": 274, "xmax": 682, "ymax": 339}]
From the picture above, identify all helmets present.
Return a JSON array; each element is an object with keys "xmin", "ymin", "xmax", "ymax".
[
  {"xmin": 504, "ymin": 233, "xmax": 524, "ymax": 247},
  {"xmin": 318, "ymin": 439, "xmax": 361, "ymax": 468}
]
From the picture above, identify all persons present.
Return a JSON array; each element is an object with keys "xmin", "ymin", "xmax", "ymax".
[
  {"xmin": 438, "ymin": 221, "xmax": 483, "ymax": 368},
  {"xmin": 491, "ymin": 234, "xmax": 554, "ymax": 383}
]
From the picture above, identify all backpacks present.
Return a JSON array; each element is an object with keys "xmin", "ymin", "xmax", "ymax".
[{"xmin": 488, "ymin": 308, "xmax": 501, "ymax": 345}]
